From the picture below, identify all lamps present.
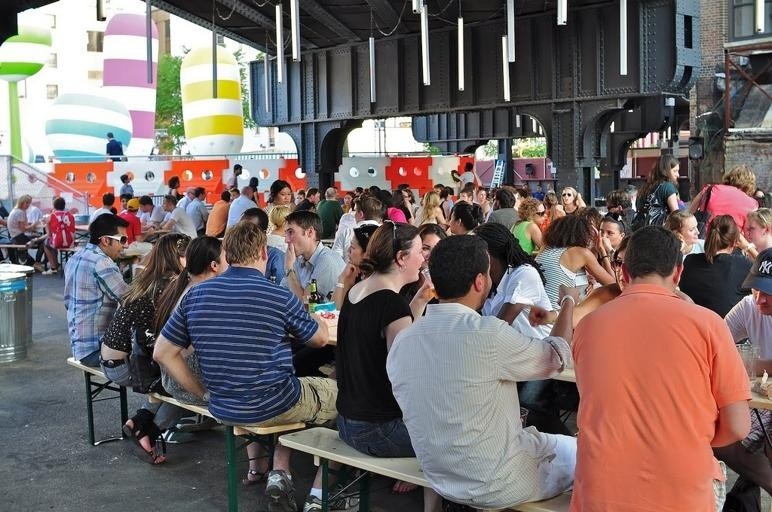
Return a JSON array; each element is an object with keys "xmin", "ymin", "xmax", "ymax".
[
  {"xmin": 458, "ymin": 17, "xmax": 464, "ymax": 91},
  {"xmin": 146, "ymin": 1, "xmax": 152, "ymax": 83},
  {"xmin": 557, "ymin": 0, "xmax": 567, "ymax": 26},
  {"xmin": 502, "ymin": 35, "xmax": 511, "ymax": 102},
  {"xmin": 508, "ymin": 0, "xmax": 515, "ymax": 63},
  {"xmin": 413, "ymin": 0, "xmax": 422, "ymax": 13},
  {"xmin": 421, "ymin": 5, "xmax": 431, "ymax": 86},
  {"xmin": 370, "ymin": 37, "xmax": 376, "ymax": 102},
  {"xmin": 265, "ymin": 55, "xmax": 270, "ymax": 113},
  {"xmin": 291, "ymin": 0, "xmax": 301, "ymax": 62},
  {"xmin": 276, "ymin": 4, "xmax": 283, "ymax": 84},
  {"xmin": 212, "ymin": 30, "xmax": 218, "ymax": 98},
  {"xmin": 620, "ymin": 0, "xmax": 627, "ymax": 76}
]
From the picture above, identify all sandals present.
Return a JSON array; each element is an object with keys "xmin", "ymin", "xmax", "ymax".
[
  {"xmin": 123, "ymin": 409, "xmax": 166, "ymax": 466},
  {"xmin": 242, "ymin": 467, "xmax": 270, "ymax": 485}
]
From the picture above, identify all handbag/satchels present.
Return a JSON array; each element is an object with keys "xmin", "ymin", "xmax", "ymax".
[
  {"xmin": 693, "ymin": 184, "xmax": 713, "ymax": 240},
  {"xmin": 629, "ymin": 182, "xmax": 669, "ymax": 233}
]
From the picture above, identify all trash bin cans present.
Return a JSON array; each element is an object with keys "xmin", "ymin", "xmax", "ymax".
[
  {"xmin": 0, "ymin": 273, "xmax": 28, "ymax": 364},
  {"xmin": 0, "ymin": 263, "xmax": 35, "ymax": 346}
]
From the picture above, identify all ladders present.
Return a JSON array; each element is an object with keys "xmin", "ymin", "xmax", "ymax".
[{"xmin": 490, "ymin": 160, "xmax": 505, "ymax": 189}]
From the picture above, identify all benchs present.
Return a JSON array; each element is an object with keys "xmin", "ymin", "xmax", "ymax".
[
  {"xmin": 1, "ymin": 244, "xmax": 85, "ymax": 277},
  {"xmin": 65, "ymin": 356, "xmax": 305, "ymax": 512},
  {"xmin": 279, "ymin": 426, "xmax": 573, "ymax": 512}
]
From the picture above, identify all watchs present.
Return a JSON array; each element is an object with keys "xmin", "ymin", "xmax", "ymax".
[
  {"xmin": 561, "ymin": 295, "xmax": 576, "ymax": 306},
  {"xmin": 286, "ymin": 269, "xmax": 296, "ymax": 276}
]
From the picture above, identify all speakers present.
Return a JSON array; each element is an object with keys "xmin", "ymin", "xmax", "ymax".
[
  {"xmin": 525, "ymin": 163, "xmax": 534, "ymax": 176},
  {"xmin": 687, "ymin": 136, "xmax": 705, "ymax": 161}
]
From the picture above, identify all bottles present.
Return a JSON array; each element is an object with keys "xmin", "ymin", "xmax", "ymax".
[{"xmin": 309, "ymin": 278, "xmax": 320, "ymax": 313}]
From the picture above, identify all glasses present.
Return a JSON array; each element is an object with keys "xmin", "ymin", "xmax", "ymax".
[
  {"xmin": 536, "ymin": 210, "xmax": 546, "ymax": 217},
  {"xmin": 384, "ymin": 219, "xmax": 396, "ymax": 258},
  {"xmin": 607, "ymin": 206, "xmax": 617, "ymax": 210},
  {"xmin": 562, "ymin": 193, "xmax": 572, "ymax": 196},
  {"xmin": 98, "ymin": 235, "xmax": 128, "ymax": 245},
  {"xmin": 610, "ymin": 260, "xmax": 624, "ymax": 271}
]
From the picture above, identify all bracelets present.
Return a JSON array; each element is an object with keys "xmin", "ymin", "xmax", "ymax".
[
  {"xmin": 335, "ymin": 282, "xmax": 344, "ymax": 289},
  {"xmin": 602, "ymin": 256, "xmax": 610, "ymax": 261},
  {"xmin": 745, "ymin": 243, "xmax": 758, "ymax": 251},
  {"xmin": 551, "ymin": 310, "xmax": 560, "ymax": 317}
]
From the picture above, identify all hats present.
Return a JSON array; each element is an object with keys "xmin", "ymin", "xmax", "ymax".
[
  {"xmin": 127, "ymin": 199, "xmax": 140, "ymax": 210},
  {"xmin": 741, "ymin": 248, "xmax": 772, "ymax": 295}
]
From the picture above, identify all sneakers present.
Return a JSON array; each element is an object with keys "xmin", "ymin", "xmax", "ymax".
[
  {"xmin": 34, "ymin": 263, "xmax": 46, "ymax": 276},
  {"xmin": 303, "ymin": 485, "xmax": 360, "ymax": 512},
  {"xmin": 264, "ymin": 470, "xmax": 298, "ymax": 512},
  {"xmin": 156, "ymin": 425, "xmax": 195, "ymax": 443},
  {"xmin": 176, "ymin": 412, "xmax": 217, "ymax": 432},
  {"xmin": 42, "ymin": 268, "xmax": 57, "ymax": 275}
]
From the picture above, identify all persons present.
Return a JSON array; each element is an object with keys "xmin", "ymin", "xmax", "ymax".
[
  {"xmin": 562, "ymin": 235, "xmax": 694, "ymax": 343},
  {"xmin": 100, "ymin": 232, "xmax": 191, "ymax": 466},
  {"xmin": 6, "ymin": 195, "xmax": 46, "ymax": 274},
  {"xmin": 414, "ymin": 191, "xmax": 447, "ymax": 226},
  {"xmin": 598, "ymin": 211, "xmax": 627, "ymax": 256},
  {"xmin": 160, "ymin": 165, "xmax": 416, "ymax": 222},
  {"xmin": 533, "ymin": 185, "xmax": 545, "ymax": 200},
  {"xmin": 63, "ymin": 214, "xmax": 221, "ymax": 443},
  {"xmin": 689, "ymin": 166, "xmax": 759, "ymax": 239},
  {"xmin": 453, "ymin": 162, "xmax": 475, "ymax": 190},
  {"xmin": 232, "ymin": 208, "xmax": 286, "ymax": 285},
  {"xmin": 135, "ymin": 195, "xmax": 165, "ymax": 230},
  {"xmin": 467, "ymin": 222, "xmax": 556, "ymax": 413},
  {"xmin": 333, "ymin": 224, "xmax": 383, "ymax": 311},
  {"xmin": 475, "ymin": 187, "xmax": 490, "ymax": 213},
  {"xmin": 679, "ymin": 215, "xmax": 758, "ymax": 320},
  {"xmin": 510, "ymin": 196, "xmax": 544, "ymax": 256},
  {"xmin": 120, "ymin": 194, "xmax": 132, "ymax": 213},
  {"xmin": 438, "ymin": 186, "xmax": 455, "ymax": 219},
  {"xmin": 120, "ymin": 175, "xmax": 133, "ymax": 196},
  {"xmin": 0, "ymin": 215, "xmax": 7, "ymax": 262},
  {"xmin": 722, "ymin": 247, "xmax": 772, "ymax": 498},
  {"xmin": 434, "ymin": 184, "xmax": 445, "ymax": 195},
  {"xmin": 745, "ymin": 207, "xmax": 772, "ymax": 251},
  {"xmin": 636, "ymin": 155, "xmax": 682, "ymax": 230},
  {"xmin": 334, "ymin": 220, "xmax": 443, "ymax": 511},
  {"xmin": 463, "ymin": 182, "xmax": 480, "ymax": 205},
  {"xmin": 414, "ymin": 225, "xmax": 445, "ymax": 271},
  {"xmin": 105, "ymin": 133, "xmax": 125, "ymax": 161},
  {"xmin": 89, "ymin": 193, "xmax": 114, "ymax": 228},
  {"xmin": 42, "ymin": 198, "xmax": 76, "ymax": 275},
  {"xmin": 157, "ymin": 235, "xmax": 228, "ymax": 407},
  {"xmin": 576, "ymin": 207, "xmax": 601, "ymax": 230},
  {"xmin": 515, "ymin": 188, "xmax": 528, "ymax": 209},
  {"xmin": 543, "ymin": 183, "xmax": 555, "ymax": 202},
  {"xmin": 450, "ymin": 188, "xmax": 483, "ymax": 222},
  {"xmin": 387, "ymin": 234, "xmax": 578, "ymax": 508},
  {"xmin": 517, "ymin": 183, "xmax": 529, "ymax": 198},
  {"xmin": 606, "ymin": 189, "xmax": 633, "ymax": 217},
  {"xmin": 284, "ymin": 210, "xmax": 342, "ymax": 300},
  {"xmin": 118, "ymin": 199, "xmax": 155, "ymax": 278},
  {"xmin": 537, "ymin": 192, "xmax": 567, "ymax": 221},
  {"xmin": 560, "ymin": 187, "xmax": 586, "ymax": 215},
  {"xmin": 449, "ymin": 200, "xmax": 485, "ymax": 237},
  {"xmin": 488, "ymin": 189, "xmax": 520, "ymax": 231},
  {"xmin": 568, "ymin": 225, "xmax": 752, "ymax": 512},
  {"xmin": 533, "ymin": 215, "xmax": 619, "ymax": 308},
  {"xmin": 26, "ymin": 195, "xmax": 42, "ymax": 231}
]
